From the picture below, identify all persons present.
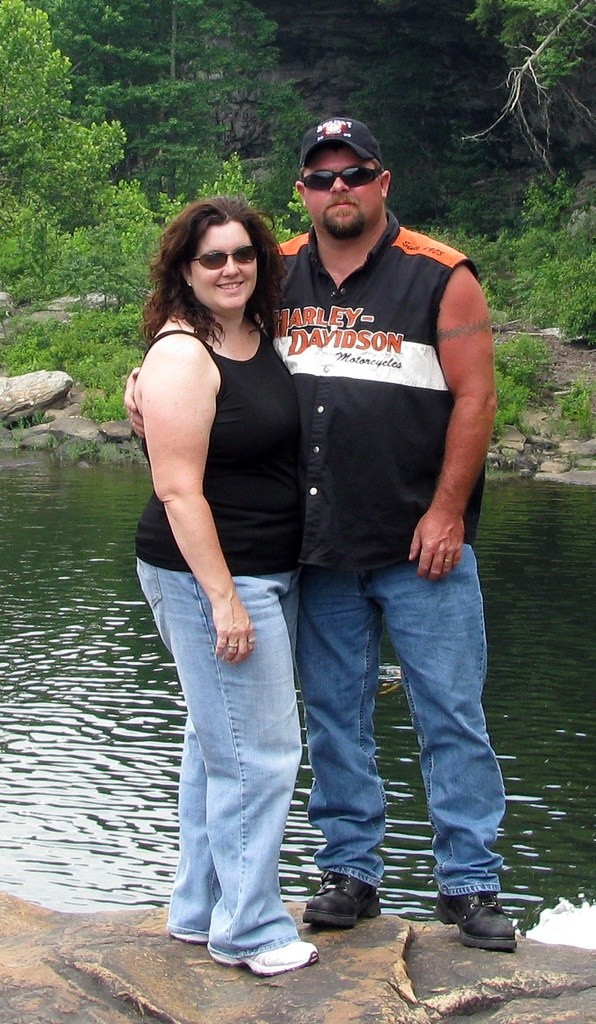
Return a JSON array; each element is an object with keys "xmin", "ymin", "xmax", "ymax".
[{"xmin": 124, "ymin": 117, "xmax": 518, "ymax": 978}]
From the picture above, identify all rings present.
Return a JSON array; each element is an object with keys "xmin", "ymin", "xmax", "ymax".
[
  {"xmin": 249, "ymin": 637, "xmax": 256, "ymax": 643},
  {"xmin": 443, "ymin": 558, "xmax": 453, "ymax": 567},
  {"xmin": 226, "ymin": 643, "xmax": 238, "ymax": 648}
]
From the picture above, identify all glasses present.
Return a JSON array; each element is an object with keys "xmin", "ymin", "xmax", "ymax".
[
  {"xmin": 298, "ymin": 166, "xmax": 389, "ymax": 191},
  {"xmin": 184, "ymin": 245, "xmax": 259, "ymax": 270}
]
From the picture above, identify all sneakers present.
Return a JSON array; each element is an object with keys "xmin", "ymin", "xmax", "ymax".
[
  {"xmin": 169, "ymin": 932, "xmax": 208, "ymax": 944},
  {"xmin": 207, "ymin": 941, "xmax": 319, "ymax": 977}
]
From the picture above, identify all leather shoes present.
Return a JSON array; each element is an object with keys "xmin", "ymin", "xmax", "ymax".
[
  {"xmin": 302, "ymin": 870, "xmax": 381, "ymax": 926},
  {"xmin": 433, "ymin": 890, "xmax": 517, "ymax": 950}
]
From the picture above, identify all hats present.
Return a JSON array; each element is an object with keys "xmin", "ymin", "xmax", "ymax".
[{"xmin": 299, "ymin": 116, "xmax": 383, "ymax": 167}]
